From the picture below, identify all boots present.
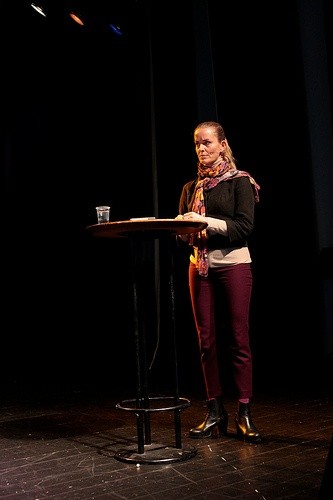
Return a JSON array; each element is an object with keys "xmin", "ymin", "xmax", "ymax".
[
  {"xmin": 234, "ymin": 401, "xmax": 261, "ymax": 441},
  {"xmin": 188, "ymin": 399, "xmax": 229, "ymax": 437}
]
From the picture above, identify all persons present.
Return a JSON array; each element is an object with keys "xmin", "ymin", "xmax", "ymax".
[{"xmin": 175, "ymin": 121, "xmax": 261, "ymax": 442}]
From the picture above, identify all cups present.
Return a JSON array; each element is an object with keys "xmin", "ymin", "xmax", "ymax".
[{"xmin": 96, "ymin": 206, "xmax": 110, "ymax": 223}]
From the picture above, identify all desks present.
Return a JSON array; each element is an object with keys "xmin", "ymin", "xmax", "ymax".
[{"xmin": 84, "ymin": 218, "xmax": 208, "ymax": 465}]
